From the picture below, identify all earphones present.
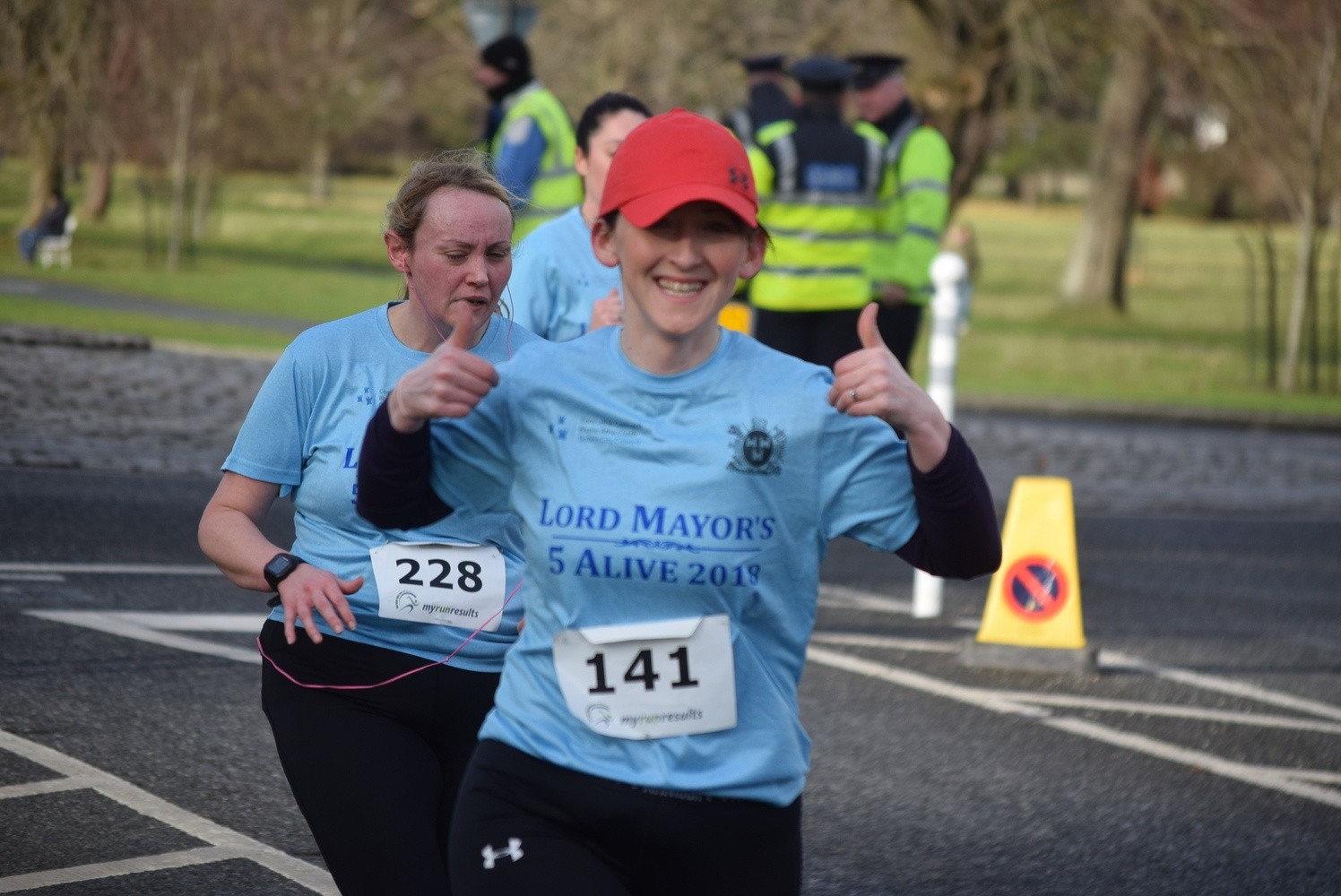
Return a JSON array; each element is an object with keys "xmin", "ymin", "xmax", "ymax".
[{"xmin": 400, "ymin": 253, "xmax": 406, "ymax": 262}]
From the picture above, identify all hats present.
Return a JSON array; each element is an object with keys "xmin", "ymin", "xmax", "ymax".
[
  {"xmin": 599, "ymin": 108, "xmax": 759, "ymax": 228},
  {"xmin": 742, "ymin": 54, "xmax": 792, "ymax": 77},
  {"xmin": 791, "ymin": 59, "xmax": 850, "ymax": 90},
  {"xmin": 484, "ymin": 33, "xmax": 528, "ymax": 74},
  {"xmin": 851, "ymin": 55, "xmax": 907, "ymax": 87}
]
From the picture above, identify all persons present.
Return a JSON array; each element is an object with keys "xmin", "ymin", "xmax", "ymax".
[
  {"xmin": 199, "ymin": 148, "xmax": 554, "ymax": 896},
  {"xmin": 352, "ymin": 108, "xmax": 1002, "ymax": 896},
  {"xmin": 16, "ymin": 189, "xmax": 70, "ymax": 266},
  {"xmin": 693, "ymin": 53, "xmax": 954, "ymax": 440},
  {"xmin": 499, "ymin": 92, "xmax": 654, "ymax": 344},
  {"xmin": 474, "ymin": 34, "xmax": 587, "ymax": 253}
]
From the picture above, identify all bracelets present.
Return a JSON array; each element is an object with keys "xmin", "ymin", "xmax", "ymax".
[{"xmin": 396, "ymin": 374, "xmax": 425, "ymax": 420}]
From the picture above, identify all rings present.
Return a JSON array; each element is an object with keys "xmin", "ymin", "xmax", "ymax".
[{"xmin": 849, "ymin": 387, "xmax": 860, "ymax": 402}]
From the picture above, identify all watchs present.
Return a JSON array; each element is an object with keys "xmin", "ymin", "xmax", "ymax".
[{"xmin": 264, "ymin": 552, "xmax": 309, "ymax": 609}]
